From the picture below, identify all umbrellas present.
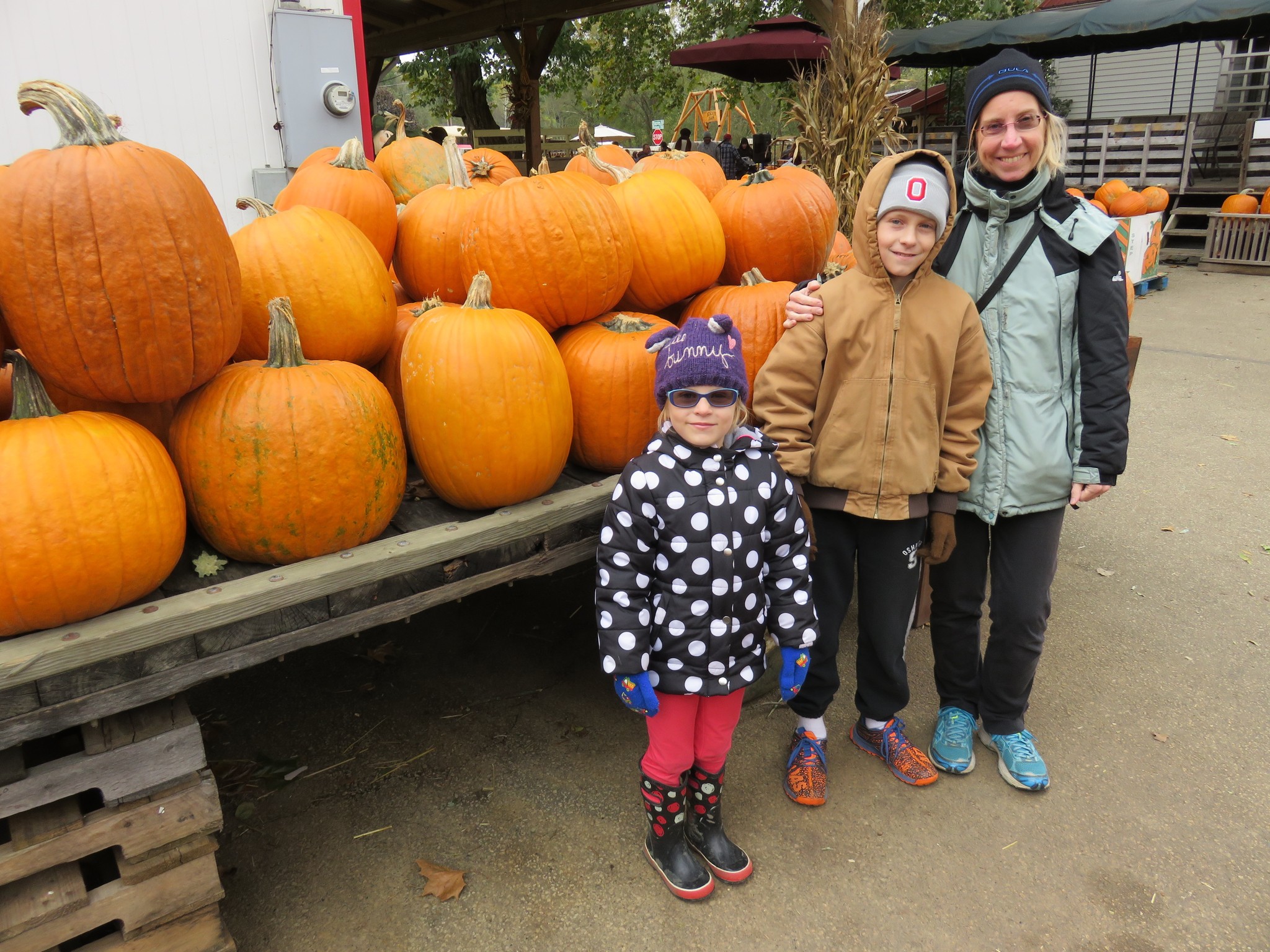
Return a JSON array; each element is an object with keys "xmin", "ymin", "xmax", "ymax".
[
  {"xmin": 668, "ymin": 16, "xmax": 901, "ymax": 84},
  {"xmin": 569, "ymin": 124, "xmax": 635, "ymax": 145}
]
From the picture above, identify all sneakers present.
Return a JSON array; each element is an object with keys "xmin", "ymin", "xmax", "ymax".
[
  {"xmin": 850, "ymin": 713, "xmax": 938, "ymax": 786},
  {"xmin": 783, "ymin": 726, "xmax": 828, "ymax": 806},
  {"xmin": 927, "ymin": 705, "xmax": 978, "ymax": 774},
  {"xmin": 978, "ymin": 722, "xmax": 1051, "ymax": 791}
]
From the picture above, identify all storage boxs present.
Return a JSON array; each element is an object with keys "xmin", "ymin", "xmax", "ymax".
[{"xmin": 1111, "ymin": 211, "xmax": 1164, "ymax": 282}]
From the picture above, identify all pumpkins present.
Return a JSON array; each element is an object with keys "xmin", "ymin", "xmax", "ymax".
[
  {"xmin": 1, "ymin": 78, "xmax": 858, "ymax": 641},
  {"xmin": 1221, "ymin": 188, "xmax": 1258, "ymax": 226},
  {"xmin": 1260, "ymin": 186, "xmax": 1270, "ymax": 229},
  {"xmin": 1065, "ymin": 180, "xmax": 1169, "ymax": 217}
]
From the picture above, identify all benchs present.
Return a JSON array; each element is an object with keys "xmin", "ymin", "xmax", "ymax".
[{"xmin": 1110, "ymin": 110, "xmax": 1260, "ymax": 179}]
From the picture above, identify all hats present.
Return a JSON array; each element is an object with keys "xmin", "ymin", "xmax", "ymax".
[
  {"xmin": 723, "ymin": 134, "xmax": 731, "ymax": 140},
  {"xmin": 963, "ymin": 48, "xmax": 1053, "ymax": 140},
  {"xmin": 876, "ymin": 162, "xmax": 950, "ymax": 242},
  {"xmin": 703, "ymin": 131, "xmax": 712, "ymax": 138},
  {"xmin": 644, "ymin": 313, "xmax": 749, "ymax": 412}
]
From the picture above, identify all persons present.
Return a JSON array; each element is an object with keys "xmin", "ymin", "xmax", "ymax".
[
  {"xmin": 593, "ymin": 313, "xmax": 819, "ymax": 902},
  {"xmin": 612, "ymin": 129, "xmax": 802, "ymax": 181},
  {"xmin": 780, "ymin": 49, "xmax": 1131, "ymax": 792},
  {"xmin": 751, "ymin": 148, "xmax": 992, "ymax": 807}
]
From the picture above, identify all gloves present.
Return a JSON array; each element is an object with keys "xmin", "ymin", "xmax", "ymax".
[
  {"xmin": 780, "ymin": 645, "xmax": 811, "ymax": 703},
  {"xmin": 913, "ymin": 512, "xmax": 957, "ymax": 566},
  {"xmin": 798, "ymin": 493, "xmax": 819, "ymax": 566},
  {"xmin": 613, "ymin": 670, "xmax": 659, "ymax": 717}
]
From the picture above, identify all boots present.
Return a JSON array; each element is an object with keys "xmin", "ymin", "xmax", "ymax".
[
  {"xmin": 683, "ymin": 761, "xmax": 753, "ymax": 885},
  {"xmin": 638, "ymin": 753, "xmax": 715, "ymax": 900}
]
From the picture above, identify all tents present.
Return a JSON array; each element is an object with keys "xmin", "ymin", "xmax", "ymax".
[{"xmin": 877, "ymin": 0, "xmax": 1270, "ymax": 187}]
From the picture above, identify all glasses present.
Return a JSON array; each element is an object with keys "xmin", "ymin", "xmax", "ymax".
[
  {"xmin": 975, "ymin": 114, "xmax": 1048, "ymax": 138},
  {"xmin": 664, "ymin": 388, "xmax": 740, "ymax": 408}
]
